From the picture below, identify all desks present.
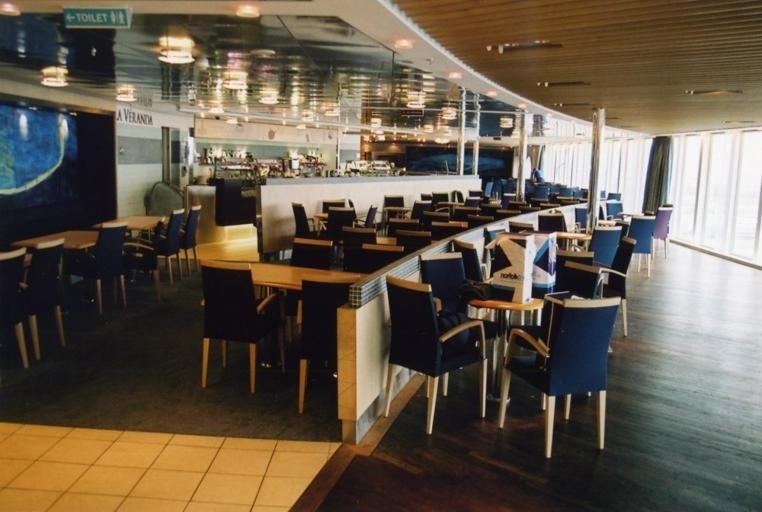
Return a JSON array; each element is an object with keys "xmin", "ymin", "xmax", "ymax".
[
  {"xmin": 217, "ymin": 259, "xmax": 371, "ymax": 381},
  {"xmin": 467, "ymin": 292, "xmax": 549, "ymax": 407}
]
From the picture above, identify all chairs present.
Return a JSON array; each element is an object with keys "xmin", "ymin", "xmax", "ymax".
[
  {"xmin": 288, "ymin": 178, "xmax": 675, "ymax": 337},
  {"xmin": 196, "ymin": 260, "xmax": 278, "ymax": 392},
  {"xmin": 293, "ymin": 278, "xmax": 357, "ymax": 419},
  {"xmin": 416, "ymin": 248, "xmax": 494, "ymax": 398},
  {"xmin": 497, "ymin": 293, "xmax": 625, "ymax": 458},
  {"xmin": 0, "ymin": 202, "xmax": 208, "ymax": 372},
  {"xmin": 379, "ymin": 273, "xmax": 491, "ymax": 436},
  {"xmin": 503, "ymin": 261, "xmax": 606, "ymax": 352}
]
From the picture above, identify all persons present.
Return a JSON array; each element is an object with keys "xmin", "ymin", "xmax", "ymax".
[{"xmin": 532, "ymin": 168, "xmax": 545, "ymax": 182}]
